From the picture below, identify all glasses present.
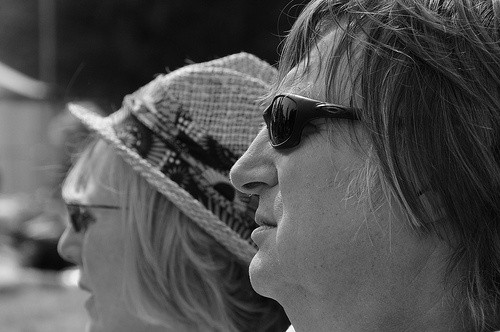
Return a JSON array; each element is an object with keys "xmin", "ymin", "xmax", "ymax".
[
  {"xmin": 261, "ymin": 92, "xmax": 365, "ymax": 156},
  {"xmin": 65, "ymin": 201, "xmax": 126, "ymax": 232}
]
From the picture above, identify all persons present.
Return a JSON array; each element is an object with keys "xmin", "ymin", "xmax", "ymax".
[
  {"xmin": 57, "ymin": 50, "xmax": 292, "ymax": 332},
  {"xmin": 230, "ymin": 0, "xmax": 499, "ymax": 332}
]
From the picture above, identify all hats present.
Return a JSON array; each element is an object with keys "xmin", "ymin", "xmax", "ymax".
[{"xmin": 65, "ymin": 53, "xmax": 279, "ymax": 262}]
